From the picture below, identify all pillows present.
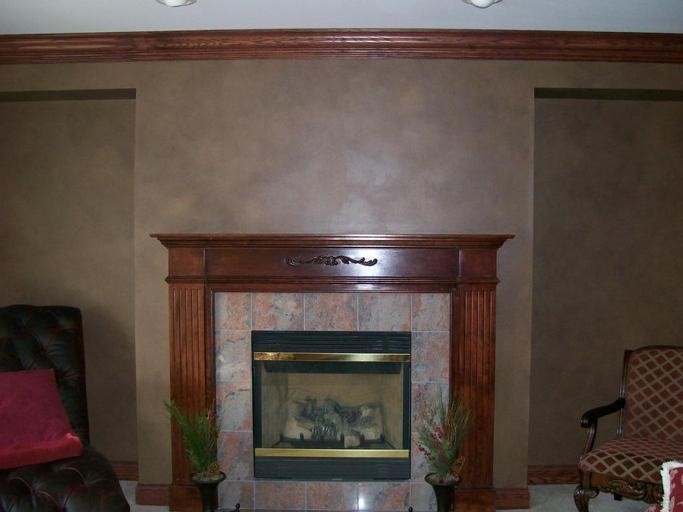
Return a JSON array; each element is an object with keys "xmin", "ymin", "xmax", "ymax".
[{"xmin": 0, "ymin": 367, "xmax": 86, "ymax": 471}]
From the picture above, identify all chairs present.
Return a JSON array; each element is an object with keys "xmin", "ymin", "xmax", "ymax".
[
  {"xmin": 571, "ymin": 344, "xmax": 683, "ymax": 511},
  {"xmin": 0, "ymin": 302, "xmax": 130, "ymax": 512}
]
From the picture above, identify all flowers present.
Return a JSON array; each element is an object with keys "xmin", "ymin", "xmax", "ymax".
[
  {"xmin": 164, "ymin": 386, "xmax": 251, "ymax": 480},
  {"xmin": 405, "ymin": 385, "xmax": 475, "ymax": 482}
]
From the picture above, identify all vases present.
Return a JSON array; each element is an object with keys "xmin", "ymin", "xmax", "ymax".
[
  {"xmin": 424, "ymin": 473, "xmax": 463, "ymax": 512},
  {"xmin": 189, "ymin": 470, "xmax": 227, "ymax": 512}
]
